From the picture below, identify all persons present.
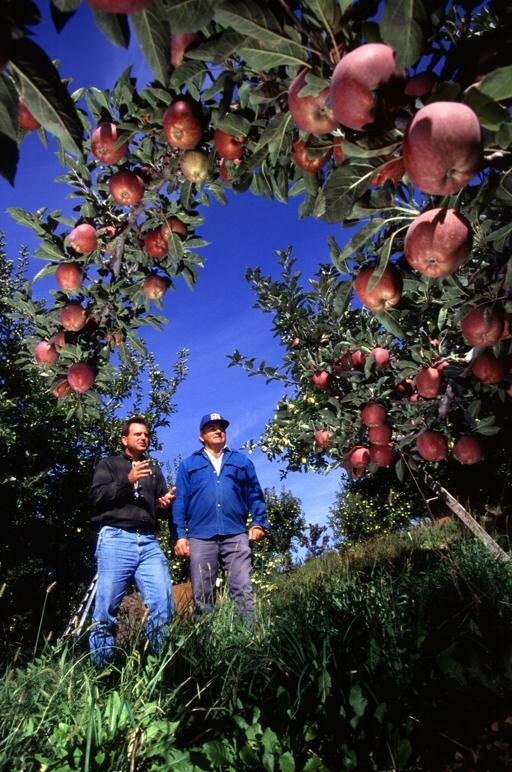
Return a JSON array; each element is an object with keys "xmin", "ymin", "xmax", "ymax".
[
  {"xmin": 88, "ymin": 416, "xmax": 176, "ymax": 671},
  {"xmin": 168, "ymin": 413, "xmax": 270, "ymax": 629}
]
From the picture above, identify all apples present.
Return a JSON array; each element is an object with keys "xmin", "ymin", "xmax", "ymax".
[
  {"xmin": 17, "ymin": 0, "xmax": 248, "ymax": 401},
  {"xmin": 287, "ymin": 42, "xmax": 499, "ymax": 197},
  {"xmin": 312, "ymin": 207, "xmax": 512, "ymax": 479}
]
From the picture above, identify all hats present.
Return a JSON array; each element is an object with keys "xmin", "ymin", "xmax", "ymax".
[{"xmin": 199, "ymin": 411, "xmax": 231, "ymax": 431}]
